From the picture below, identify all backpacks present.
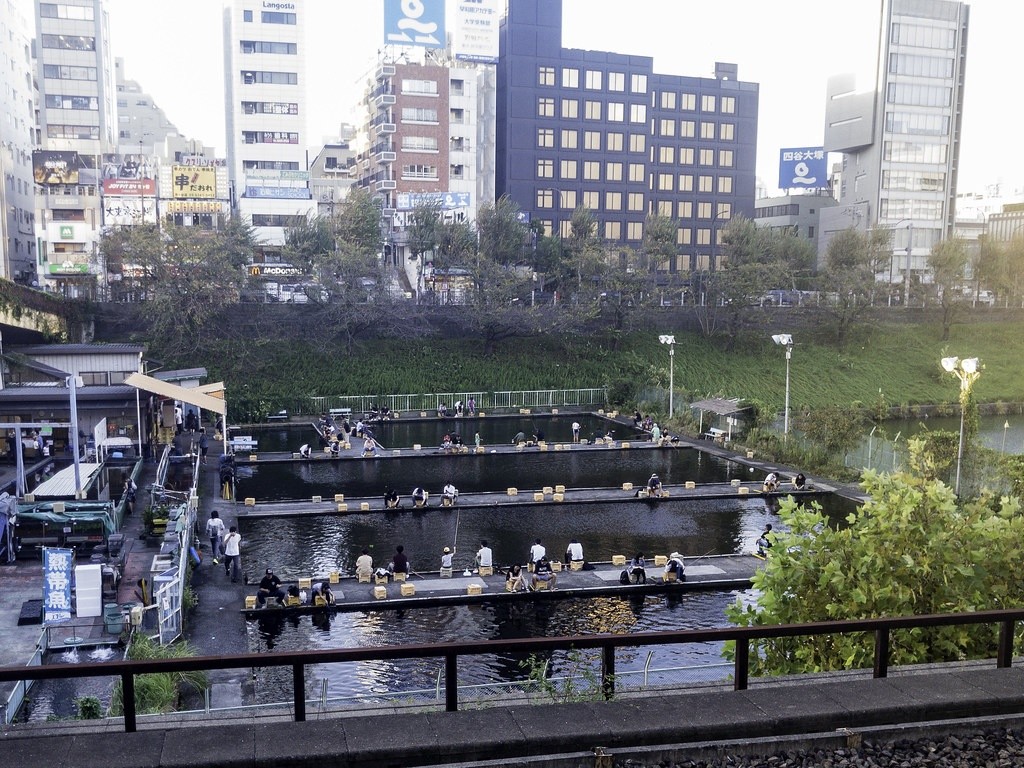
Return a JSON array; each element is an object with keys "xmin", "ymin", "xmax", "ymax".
[{"xmin": 210, "ymin": 524, "xmax": 219, "ymax": 537}]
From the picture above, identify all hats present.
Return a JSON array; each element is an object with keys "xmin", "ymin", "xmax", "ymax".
[
  {"xmin": 651, "ymin": 473, "xmax": 659, "ymax": 479},
  {"xmin": 541, "ymin": 556, "xmax": 549, "ymax": 562},
  {"xmin": 444, "ymin": 547, "xmax": 450, "ymax": 553}
]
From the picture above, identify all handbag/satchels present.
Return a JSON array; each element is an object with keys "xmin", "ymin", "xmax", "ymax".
[{"xmin": 619, "ymin": 570, "xmax": 630, "ymax": 585}]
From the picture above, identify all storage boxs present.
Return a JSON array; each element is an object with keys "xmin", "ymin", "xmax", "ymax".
[
  {"xmin": 243, "ymin": 434, "xmax": 631, "ymax": 461},
  {"xmin": 157, "ymin": 426, "xmax": 175, "ymax": 444},
  {"xmin": 244, "ymin": 552, "xmax": 686, "ymax": 611},
  {"xmin": 75, "ymin": 565, "xmax": 103, "ymax": 618},
  {"xmin": 598, "ymin": 409, "xmax": 618, "ymax": 418},
  {"xmin": 244, "ymin": 476, "xmax": 814, "ymax": 514},
  {"xmin": 364, "ymin": 408, "xmax": 560, "ymax": 420},
  {"xmin": 747, "ymin": 452, "xmax": 754, "ymax": 460}
]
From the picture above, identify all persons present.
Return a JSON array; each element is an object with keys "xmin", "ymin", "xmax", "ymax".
[
  {"xmin": 563, "ymin": 538, "xmax": 583, "ymax": 571},
  {"xmin": 763, "ymin": 472, "xmax": 780, "ymax": 492},
  {"xmin": 629, "ymin": 552, "xmax": 647, "ymax": 584},
  {"xmin": 3, "ymin": 401, "xmax": 225, "ymax": 510},
  {"xmin": 387, "ymin": 544, "xmax": 410, "ymax": 578},
  {"xmin": 224, "ymin": 525, "xmax": 241, "ymax": 581},
  {"xmin": 647, "ymin": 473, "xmax": 662, "ymax": 498},
  {"xmin": 529, "ymin": 538, "xmax": 546, "ymax": 565},
  {"xmin": 506, "ymin": 563, "xmax": 530, "ymax": 593},
  {"xmin": 384, "ymin": 488, "xmax": 401, "ymax": 509},
  {"xmin": 794, "ymin": 473, "xmax": 806, "ymax": 491},
  {"xmin": 412, "ymin": 487, "xmax": 429, "ymax": 508},
  {"xmin": 355, "ymin": 548, "xmax": 373, "ymax": 579},
  {"xmin": 439, "ymin": 480, "xmax": 455, "ymax": 507},
  {"xmin": 205, "ymin": 511, "xmax": 224, "ymax": 563},
  {"xmin": 258, "ymin": 568, "xmax": 284, "ymax": 608},
  {"xmin": 472, "ymin": 539, "xmax": 492, "ymax": 573},
  {"xmin": 664, "ymin": 557, "xmax": 685, "ymax": 583},
  {"xmin": 441, "ymin": 545, "xmax": 456, "ymax": 568},
  {"xmin": 756, "ymin": 523, "xmax": 779, "ymax": 555},
  {"xmin": 530, "ymin": 556, "xmax": 559, "ymax": 590},
  {"xmin": 310, "ymin": 583, "xmax": 337, "ymax": 606},
  {"xmin": 295, "ymin": 395, "xmax": 671, "ymax": 458}
]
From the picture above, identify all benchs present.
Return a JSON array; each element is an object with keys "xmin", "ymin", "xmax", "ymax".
[
  {"xmin": 704, "ymin": 427, "xmax": 727, "ymax": 442},
  {"xmin": 24, "ymin": 447, "xmax": 35, "ymax": 457},
  {"xmin": 267, "ymin": 410, "xmax": 287, "ymax": 422},
  {"xmin": 329, "ymin": 408, "xmax": 352, "ymax": 421}
]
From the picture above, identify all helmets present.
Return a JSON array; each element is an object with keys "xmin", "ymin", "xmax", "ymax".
[{"xmin": 265, "ymin": 568, "xmax": 273, "ymax": 575}]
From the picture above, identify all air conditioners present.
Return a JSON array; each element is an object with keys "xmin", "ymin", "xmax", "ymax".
[{"xmin": 66, "ymin": 376, "xmax": 84, "ymax": 388}]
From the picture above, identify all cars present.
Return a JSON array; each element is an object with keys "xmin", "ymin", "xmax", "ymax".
[{"xmin": 238, "ymin": 290, "xmax": 280, "ymax": 303}]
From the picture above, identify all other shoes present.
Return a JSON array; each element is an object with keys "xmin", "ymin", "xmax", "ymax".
[
  {"xmin": 448, "ymin": 503, "xmax": 452, "ymax": 507},
  {"xmin": 226, "ymin": 570, "xmax": 231, "ymax": 576},
  {"xmin": 412, "ymin": 505, "xmax": 417, "ymax": 508},
  {"xmin": 262, "ymin": 601, "xmax": 267, "ymax": 609},
  {"xmin": 212, "ymin": 559, "xmax": 218, "ymax": 565},
  {"xmin": 439, "ymin": 503, "xmax": 443, "ymax": 507},
  {"xmin": 525, "ymin": 588, "xmax": 530, "ymax": 593},
  {"xmin": 201, "ymin": 459, "xmax": 207, "ymax": 466},
  {"xmin": 423, "ymin": 505, "xmax": 427, "ymax": 508},
  {"xmin": 551, "ymin": 585, "xmax": 559, "ymax": 590},
  {"xmin": 473, "ymin": 569, "xmax": 479, "ymax": 573},
  {"xmin": 511, "ymin": 588, "xmax": 517, "ymax": 593},
  {"xmin": 666, "ymin": 577, "xmax": 669, "ymax": 581},
  {"xmin": 636, "ymin": 580, "xmax": 640, "ymax": 584},
  {"xmin": 276, "ymin": 601, "xmax": 282, "ymax": 607},
  {"xmin": 758, "ymin": 550, "xmax": 764, "ymax": 555},
  {"xmin": 676, "ymin": 578, "xmax": 683, "ymax": 583}
]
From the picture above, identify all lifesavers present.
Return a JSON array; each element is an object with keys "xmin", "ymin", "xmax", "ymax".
[{"xmin": 190, "ymin": 547, "xmax": 201, "ymax": 564}]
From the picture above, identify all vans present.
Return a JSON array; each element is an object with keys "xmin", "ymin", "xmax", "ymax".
[
  {"xmin": 749, "ymin": 290, "xmax": 799, "ymax": 307},
  {"xmin": 279, "ymin": 284, "xmax": 328, "ymax": 303}
]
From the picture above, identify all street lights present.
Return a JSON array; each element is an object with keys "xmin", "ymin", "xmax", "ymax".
[
  {"xmin": 659, "ymin": 334, "xmax": 677, "ymax": 420},
  {"xmin": 941, "ymin": 352, "xmax": 977, "ymax": 501},
  {"xmin": 772, "ymin": 331, "xmax": 793, "ymax": 447}
]
